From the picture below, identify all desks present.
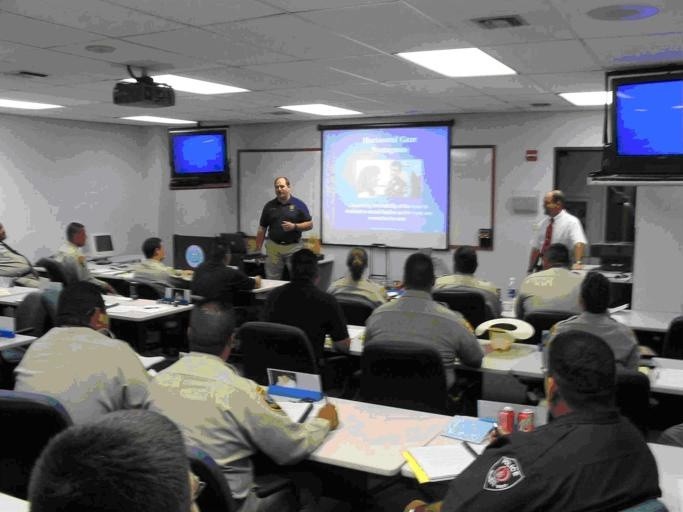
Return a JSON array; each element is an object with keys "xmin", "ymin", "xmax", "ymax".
[
  {"xmin": 513, "ymin": 344, "xmax": 683, "ymax": 396},
  {"xmin": 244, "ymin": 249, "xmax": 334, "ymax": 292},
  {"xmin": 257, "ymin": 384, "xmax": 453, "ymax": 478},
  {"xmin": 0, "ymin": 285, "xmax": 40, "ymax": 318},
  {"xmin": 240, "ymin": 275, "xmax": 291, "ymax": 300},
  {"xmin": 575, "ymin": 261, "xmax": 631, "ymax": 308},
  {"xmin": 138, "ymin": 355, "xmax": 165, "ymax": 373},
  {"xmin": 98, "ymin": 293, "xmax": 194, "ymax": 350},
  {"xmin": 400, "ymin": 415, "xmax": 683, "ymax": 511},
  {"xmin": 0, "ymin": 326, "xmax": 36, "ymax": 349},
  {"xmin": 87, "ymin": 253, "xmax": 137, "ymax": 295},
  {"xmin": 502, "ymin": 305, "xmax": 681, "ymax": 334},
  {"xmin": 333, "ymin": 321, "xmax": 541, "ymax": 377}
]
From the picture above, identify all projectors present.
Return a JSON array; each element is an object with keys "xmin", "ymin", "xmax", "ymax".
[{"xmin": 113, "ymin": 77, "xmax": 176, "ymax": 108}]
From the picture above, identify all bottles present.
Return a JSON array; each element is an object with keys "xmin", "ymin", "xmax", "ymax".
[{"xmin": 507, "ymin": 277, "xmax": 516, "ymax": 302}]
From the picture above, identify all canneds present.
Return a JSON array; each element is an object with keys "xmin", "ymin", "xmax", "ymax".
[
  {"xmin": 518, "ymin": 409, "xmax": 535, "ymax": 432},
  {"xmin": 498, "ymin": 407, "xmax": 514, "ymax": 434}
]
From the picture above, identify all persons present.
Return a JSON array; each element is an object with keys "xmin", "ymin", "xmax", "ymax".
[
  {"xmin": 13, "ymin": 281, "xmax": 152, "ymax": 426},
  {"xmin": 431, "ymin": 246, "xmax": 502, "ymax": 318},
  {"xmin": 48, "ymin": 222, "xmax": 118, "ymax": 295},
  {"xmin": 142, "ymin": 300, "xmax": 340, "ymax": 512},
  {"xmin": 527, "ymin": 189, "xmax": 588, "ymax": 275},
  {"xmin": 0, "ymin": 222, "xmax": 49, "ymax": 289},
  {"xmin": 133, "ymin": 237, "xmax": 193, "ymax": 352},
  {"xmin": 514, "ymin": 243, "xmax": 611, "ymax": 320},
  {"xmin": 258, "ymin": 249, "xmax": 360, "ymax": 398},
  {"xmin": 188, "ymin": 239, "xmax": 262, "ymax": 363},
  {"xmin": 357, "ymin": 164, "xmax": 380, "ymax": 199},
  {"xmin": 365, "ymin": 330, "xmax": 662, "ymax": 512},
  {"xmin": 384, "ymin": 161, "xmax": 405, "ymax": 197},
  {"xmin": 326, "ymin": 248, "xmax": 391, "ymax": 310},
  {"xmin": 363, "ymin": 253, "xmax": 485, "ymax": 411},
  {"xmin": 28, "ymin": 408, "xmax": 206, "ymax": 512},
  {"xmin": 543, "ymin": 271, "xmax": 639, "ymax": 374},
  {"xmin": 254, "ymin": 176, "xmax": 314, "ymax": 280}
]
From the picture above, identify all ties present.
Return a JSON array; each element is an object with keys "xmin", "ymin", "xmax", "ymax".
[{"xmin": 540, "ymin": 218, "xmax": 555, "ymax": 256}]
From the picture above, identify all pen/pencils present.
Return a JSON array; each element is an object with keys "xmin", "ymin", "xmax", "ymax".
[{"xmin": 144, "ymin": 305, "xmax": 161, "ymax": 310}]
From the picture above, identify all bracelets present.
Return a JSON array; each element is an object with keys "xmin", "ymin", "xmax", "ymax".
[{"xmin": 294, "ymin": 223, "xmax": 297, "ymax": 231}]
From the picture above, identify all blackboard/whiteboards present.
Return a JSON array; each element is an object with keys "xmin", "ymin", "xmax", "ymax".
[
  {"xmin": 236, "ymin": 148, "xmax": 322, "ymax": 243},
  {"xmin": 449, "ymin": 145, "xmax": 495, "ymax": 251}
]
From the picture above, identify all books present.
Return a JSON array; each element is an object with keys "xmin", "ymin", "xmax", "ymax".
[
  {"xmin": 0, "ymin": 288, "xmax": 12, "ymax": 298},
  {"xmin": 90, "ymin": 268, "xmax": 125, "ymax": 276},
  {"xmin": 440, "ymin": 414, "xmax": 497, "ymax": 444},
  {"xmin": 104, "ymin": 300, "xmax": 120, "ymax": 309}
]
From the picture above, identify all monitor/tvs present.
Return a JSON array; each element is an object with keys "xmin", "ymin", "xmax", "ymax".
[
  {"xmin": 83, "ymin": 232, "xmax": 115, "ymax": 265},
  {"xmin": 169, "ymin": 130, "xmax": 232, "ymax": 191},
  {"xmin": 603, "ymin": 63, "xmax": 683, "ymax": 176}
]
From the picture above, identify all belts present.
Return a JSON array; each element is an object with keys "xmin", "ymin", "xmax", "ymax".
[{"xmin": 270, "ymin": 238, "xmax": 299, "ymax": 246}]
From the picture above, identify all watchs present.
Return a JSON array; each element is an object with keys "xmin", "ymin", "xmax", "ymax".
[
  {"xmin": 408, "ymin": 504, "xmax": 427, "ymax": 512},
  {"xmin": 574, "ymin": 260, "xmax": 583, "ymax": 264}
]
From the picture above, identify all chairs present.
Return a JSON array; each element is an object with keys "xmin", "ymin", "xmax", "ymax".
[
  {"xmin": 135, "ymin": 282, "xmax": 162, "ymax": 356},
  {"xmin": 0, "ymin": 389, "xmax": 74, "ymax": 497},
  {"xmin": 238, "ymin": 320, "xmax": 350, "ymax": 395},
  {"xmin": 432, "ymin": 291, "xmax": 486, "ymax": 329},
  {"xmin": 361, "ymin": 342, "xmax": 477, "ymax": 417},
  {"xmin": 525, "ymin": 311, "xmax": 576, "ymax": 342},
  {"xmin": 184, "ymin": 443, "xmax": 302, "ymax": 512},
  {"xmin": 15, "ymin": 295, "xmax": 53, "ymax": 338},
  {"xmin": 659, "ymin": 316, "xmax": 683, "ymax": 357},
  {"xmin": 332, "ymin": 294, "xmax": 375, "ymax": 321}
]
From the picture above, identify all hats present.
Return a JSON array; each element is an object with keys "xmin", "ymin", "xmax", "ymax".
[{"xmin": 474, "ymin": 317, "xmax": 536, "ymax": 351}]
